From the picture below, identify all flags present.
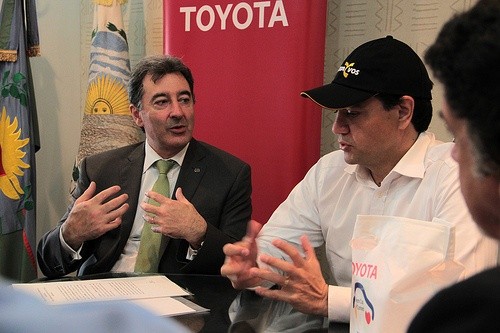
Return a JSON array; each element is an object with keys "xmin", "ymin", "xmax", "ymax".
[
  {"xmin": 0, "ymin": 0, "xmax": 41, "ymax": 283},
  {"xmin": 69, "ymin": 0, "xmax": 143, "ymax": 200}
]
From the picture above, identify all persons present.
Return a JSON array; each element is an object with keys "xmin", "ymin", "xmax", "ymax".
[
  {"xmin": 228, "ymin": 289, "xmax": 324, "ymax": 333},
  {"xmin": 37, "ymin": 54, "xmax": 252, "ymax": 275},
  {"xmin": 220, "ymin": 35, "xmax": 500, "ymax": 333},
  {"xmin": 406, "ymin": 1, "xmax": 500, "ymax": 333}
]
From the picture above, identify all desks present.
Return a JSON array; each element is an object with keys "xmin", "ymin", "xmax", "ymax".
[{"xmin": 14, "ymin": 271, "xmax": 350, "ymax": 333}]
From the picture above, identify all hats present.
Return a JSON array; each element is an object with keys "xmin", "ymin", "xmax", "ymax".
[{"xmin": 301, "ymin": 36, "xmax": 432, "ymax": 111}]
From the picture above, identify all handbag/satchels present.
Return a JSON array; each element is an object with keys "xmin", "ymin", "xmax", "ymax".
[{"xmin": 348, "ymin": 214, "xmax": 464, "ymax": 333}]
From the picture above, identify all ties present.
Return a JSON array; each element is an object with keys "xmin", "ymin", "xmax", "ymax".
[{"xmin": 134, "ymin": 160, "xmax": 176, "ymax": 273}]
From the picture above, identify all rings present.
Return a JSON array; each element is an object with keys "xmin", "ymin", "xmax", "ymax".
[
  {"xmin": 283, "ymin": 276, "xmax": 289, "ymax": 287},
  {"xmin": 152, "ymin": 216, "xmax": 155, "ymax": 224}
]
